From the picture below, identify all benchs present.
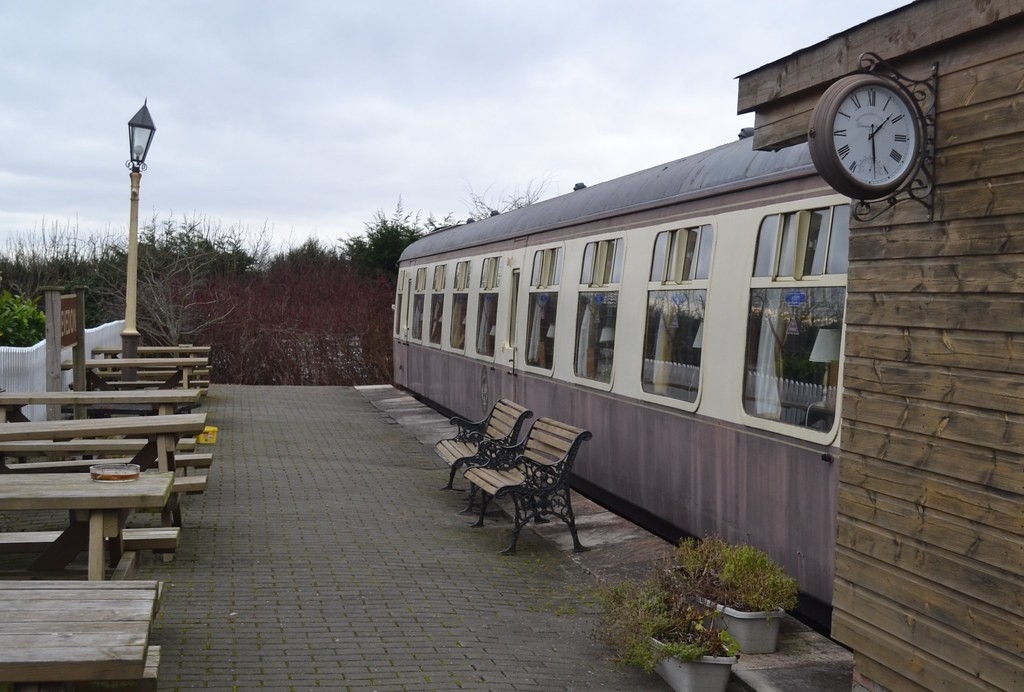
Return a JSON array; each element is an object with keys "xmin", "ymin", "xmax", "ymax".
[{"xmin": 433, "ymin": 397, "xmax": 594, "ymax": 556}]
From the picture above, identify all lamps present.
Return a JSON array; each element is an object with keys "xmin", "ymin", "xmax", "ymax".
[{"xmin": 421, "ymin": 300, "xmax": 843, "ymax": 427}]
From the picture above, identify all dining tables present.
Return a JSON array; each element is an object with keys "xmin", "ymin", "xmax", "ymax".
[{"xmin": 0, "ymin": 345, "xmax": 214, "ymax": 692}]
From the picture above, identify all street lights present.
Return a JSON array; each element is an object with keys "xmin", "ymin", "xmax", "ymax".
[{"xmin": 119, "ymin": 93, "xmax": 155, "ymax": 388}]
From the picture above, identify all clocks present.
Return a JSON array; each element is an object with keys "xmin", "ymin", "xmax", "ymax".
[{"xmin": 806, "ymin": 48, "xmax": 943, "ymax": 223}]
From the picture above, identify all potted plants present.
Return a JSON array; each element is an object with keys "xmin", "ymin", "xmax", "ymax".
[{"xmin": 606, "ymin": 536, "xmax": 798, "ymax": 690}]
